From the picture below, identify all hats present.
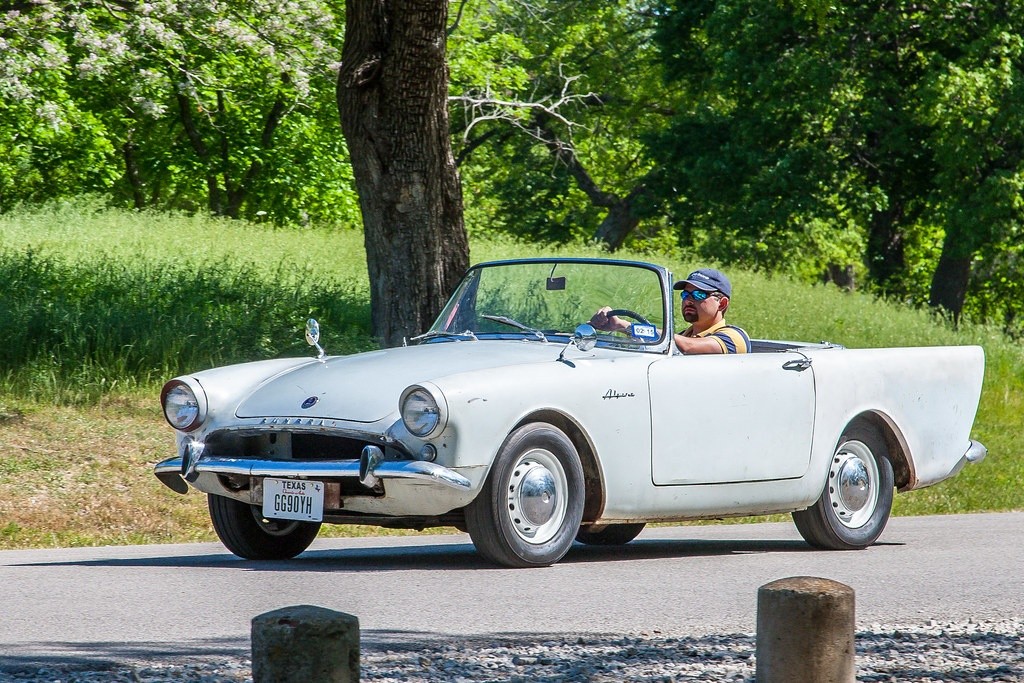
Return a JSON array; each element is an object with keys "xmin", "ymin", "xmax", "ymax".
[{"xmin": 673, "ymin": 269, "xmax": 730, "ymax": 299}]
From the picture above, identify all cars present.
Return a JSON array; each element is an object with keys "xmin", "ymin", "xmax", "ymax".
[{"xmin": 154, "ymin": 258, "xmax": 989, "ymax": 569}]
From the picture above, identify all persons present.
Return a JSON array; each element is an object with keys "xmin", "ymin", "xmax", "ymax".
[{"xmin": 586, "ymin": 269, "xmax": 752, "ymax": 355}]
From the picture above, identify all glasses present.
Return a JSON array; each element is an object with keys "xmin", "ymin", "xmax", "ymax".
[{"xmin": 681, "ymin": 291, "xmax": 722, "ymax": 301}]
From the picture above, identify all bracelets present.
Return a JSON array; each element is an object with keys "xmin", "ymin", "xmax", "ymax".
[{"xmin": 625, "ymin": 324, "xmax": 632, "ymax": 335}]
